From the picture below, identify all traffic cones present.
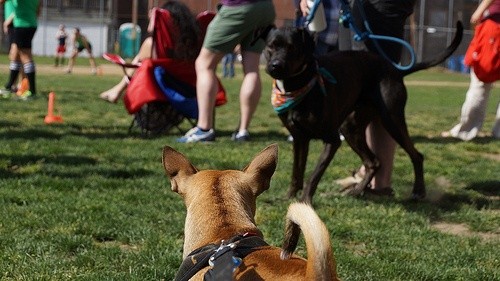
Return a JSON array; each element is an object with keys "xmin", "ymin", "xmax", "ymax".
[{"xmin": 44, "ymin": 91, "xmax": 62, "ymax": 124}]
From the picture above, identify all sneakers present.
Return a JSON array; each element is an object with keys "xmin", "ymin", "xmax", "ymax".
[
  {"xmin": 176, "ymin": 127, "xmax": 215, "ymax": 143},
  {"xmin": 231, "ymin": 128, "xmax": 250, "ymax": 143}
]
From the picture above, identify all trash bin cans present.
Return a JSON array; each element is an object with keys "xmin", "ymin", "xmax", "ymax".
[{"xmin": 446, "ymin": 54, "xmax": 470, "ymax": 73}]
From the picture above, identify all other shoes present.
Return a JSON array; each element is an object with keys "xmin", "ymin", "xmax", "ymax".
[
  {"xmin": 17, "ymin": 94, "xmax": 38, "ymax": 102},
  {"xmin": 342, "ymin": 171, "xmax": 395, "ymax": 200},
  {"xmin": 0, "ymin": 88, "xmax": 11, "ymax": 99}
]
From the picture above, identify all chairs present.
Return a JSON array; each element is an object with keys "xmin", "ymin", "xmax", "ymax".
[{"xmin": 101, "ymin": 7, "xmax": 229, "ymax": 134}]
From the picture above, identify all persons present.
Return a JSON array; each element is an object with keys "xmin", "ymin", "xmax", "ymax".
[
  {"xmin": 222, "ymin": 43, "xmax": 241, "ymax": 79},
  {"xmin": 298, "ymin": 0, "xmax": 413, "ymax": 202},
  {"xmin": 0, "ymin": 0, "xmax": 43, "ymax": 99},
  {"xmin": 53, "ymin": 24, "xmax": 68, "ymax": 68},
  {"xmin": 98, "ymin": 0, "xmax": 198, "ymax": 104},
  {"xmin": 65, "ymin": 27, "xmax": 97, "ymax": 75},
  {"xmin": 176, "ymin": 0, "xmax": 277, "ymax": 144},
  {"xmin": 430, "ymin": 0, "xmax": 500, "ymax": 141}
]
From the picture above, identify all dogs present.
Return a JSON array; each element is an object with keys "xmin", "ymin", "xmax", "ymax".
[
  {"xmin": 263, "ymin": 21, "xmax": 464, "ymax": 207},
  {"xmin": 162, "ymin": 143, "xmax": 341, "ymax": 281}
]
